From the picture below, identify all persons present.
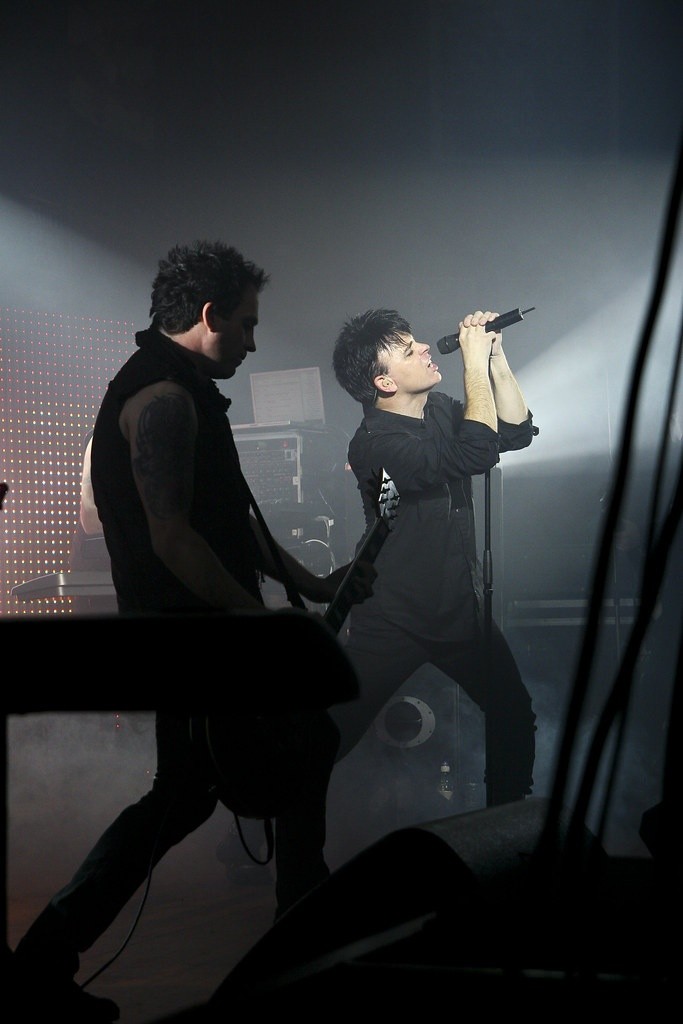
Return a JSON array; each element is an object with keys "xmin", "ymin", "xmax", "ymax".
[
  {"xmin": 0, "ymin": 240, "xmax": 377, "ymax": 1024},
  {"xmin": 314, "ymin": 306, "xmax": 537, "ymax": 806}
]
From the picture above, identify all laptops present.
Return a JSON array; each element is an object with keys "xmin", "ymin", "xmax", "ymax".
[{"xmin": 250, "ymin": 367, "xmax": 326, "ymax": 424}]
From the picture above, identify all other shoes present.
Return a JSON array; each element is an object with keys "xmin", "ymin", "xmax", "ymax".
[{"xmin": 69, "ymin": 981, "xmax": 120, "ymax": 1024}]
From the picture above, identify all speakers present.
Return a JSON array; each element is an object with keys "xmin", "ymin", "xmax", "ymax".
[{"xmin": 200, "ymin": 794, "xmax": 608, "ymax": 1024}]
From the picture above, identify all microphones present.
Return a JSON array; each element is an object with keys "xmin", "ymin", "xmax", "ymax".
[{"xmin": 436, "ymin": 307, "xmax": 536, "ymax": 355}]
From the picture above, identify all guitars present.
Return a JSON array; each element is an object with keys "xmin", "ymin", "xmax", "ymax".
[{"xmin": 186, "ymin": 461, "xmax": 403, "ymax": 822}]
